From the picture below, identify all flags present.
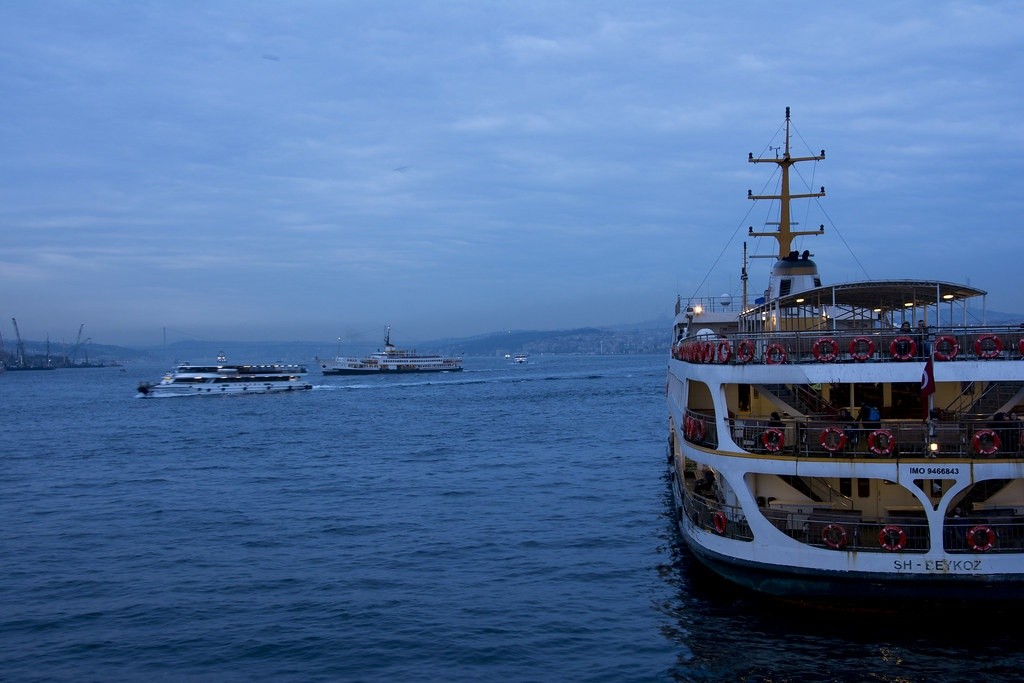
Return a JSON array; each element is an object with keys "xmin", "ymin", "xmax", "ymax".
[{"xmin": 920, "ymin": 351, "xmax": 935, "ymax": 400}]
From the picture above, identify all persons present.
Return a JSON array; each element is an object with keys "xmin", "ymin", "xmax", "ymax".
[{"xmin": 763, "ymin": 316, "xmax": 1024, "ymax": 448}]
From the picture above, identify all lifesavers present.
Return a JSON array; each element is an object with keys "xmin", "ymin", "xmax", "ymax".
[
  {"xmin": 889, "ymin": 336, "xmax": 916, "ymax": 361},
  {"xmin": 974, "ymin": 334, "xmax": 1002, "ymax": 359},
  {"xmin": 765, "ymin": 343, "xmax": 786, "ymax": 366},
  {"xmin": 764, "ymin": 428, "xmax": 785, "ymax": 452},
  {"xmin": 738, "ymin": 340, "xmax": 754, "ymax": 362},
  {"xmin": 820, "ymin": 427, "xmax": 847, "ymax": 452},
  {"xmin": 1019, "ymin": 336, "xmax": 1024, "ymax": 355},
  {"xmin": 933, "ymin": 336, "xmax": 959, "ymax": 361},
  {"xmin": 812, "ymin": 337, "xmax": 838, "ymax": 363},
  {"xmin": 972, "ymin": 428, "xmax": 1000, "ymax": 455},
  {"xmin": 868, "ymin": 428, "xmax": 895, "ymax": 456},
  {"xmin": 679, "ymin": 342, "xmax": 704, "ymax": 363},
  {"xmin": 682, "ymin": 415, "xmax": 707, "ymax": 442},
  {"xmin": 849, "ymin": 337, "xmax": 875, "ymax": 361},
  {"xmin": 967, "ymin": 525, "xmax": 995, "ymax": 553},
  {"xmin": 717, "ymin": 341, "xmax": 731, "ymax": 362},
  {"xmin": 878, "ymin": 526, "xmax": 906, "ymax": 551},
  {"xmin": 822, "ymin": 525, "xmax": 846, "ymax": 549},
  {"xmin": 713, "ymin": 511, "xmax": 726, "ymax": 534},
  {"xmin": 704, "ymin": 342, "xmax": 715, "ymax": 362}
]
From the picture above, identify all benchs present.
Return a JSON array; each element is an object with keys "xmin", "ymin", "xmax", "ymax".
[
  {"xmin": 935, "ymin": 424, "xmax": 967, "ymax": 458},
  {"xmin": 880, "ymin": 419, "xmax": 924, "ymax": 458}
]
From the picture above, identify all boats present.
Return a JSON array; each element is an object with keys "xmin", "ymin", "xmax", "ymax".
[
  {"xmin": 134, "ymin": 350, "xmax": 314, "ymax": 394},
  {"xmin": 664, "ymin": 103, "xmax": 1024, "ymax": 611},
  {"xmin": 319, "ymin": 322, "xmax": 464, "ymax": 375}
]
